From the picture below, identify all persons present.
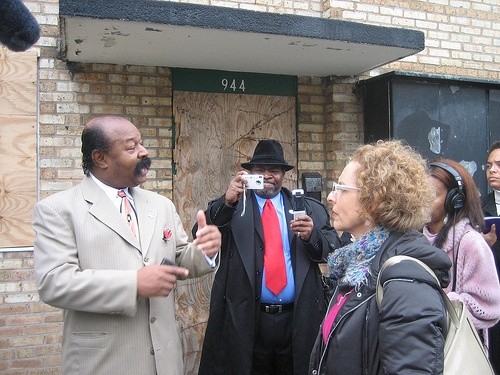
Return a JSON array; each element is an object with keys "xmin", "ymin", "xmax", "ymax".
[
  {"xmin": 191, "ymin": 139, "xmax": 342, "ymax": 375},
  {"xmin": 308, "ymin": 138, "xmax": 452, "ymax": 375},
  {"xmin": 422, "ymin": 157, "xmax": 500, "ymax": 329},
  {"xmin": 32, "ymin": 114, "xmax": 223, "ymax": 375},
  {"xmin": 475, "ymin": 140, "xmax": 500, "ymax": 375}
]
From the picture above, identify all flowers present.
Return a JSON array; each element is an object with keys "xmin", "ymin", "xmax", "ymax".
[{"xmin": 162, "ymin": 226, "xmax": 173, "ymax": 242}]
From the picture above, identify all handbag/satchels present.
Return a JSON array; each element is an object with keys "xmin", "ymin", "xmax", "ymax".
[{"xmin": 376, "ymin": 255, "xmax": 494, "ymax": 375}]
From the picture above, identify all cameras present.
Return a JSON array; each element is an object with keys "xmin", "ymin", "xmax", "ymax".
[{"xmin": 240, "ymin": 175, "xmax": 264, "ymax": 189}]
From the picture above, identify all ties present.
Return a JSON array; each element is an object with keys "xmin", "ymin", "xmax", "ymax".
[
  {"xmin": 261, "ymin": 198, "xmax": 287, "ymax": 297},
  {"xmin": 118, "ymin": 191, "xmax": 137, "ymax": 240}
]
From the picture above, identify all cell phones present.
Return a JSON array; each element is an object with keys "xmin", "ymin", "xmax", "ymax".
[{"xmin": 292, "ymin": 189, "xmax": 307, "ymax": 222}]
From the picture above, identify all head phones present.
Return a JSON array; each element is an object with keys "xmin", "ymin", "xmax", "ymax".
[{"xmin": 429, "ymin": 162, "xmax": 466, "ymax": 214}]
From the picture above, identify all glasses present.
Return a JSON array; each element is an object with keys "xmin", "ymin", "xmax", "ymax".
[
  {"xmin": 481, "ymin": 162, "xmax": 500, "ymax": 171},
  {"xmin": 332, "ymin": 181, "xmax": 359, "ymax": 194}
]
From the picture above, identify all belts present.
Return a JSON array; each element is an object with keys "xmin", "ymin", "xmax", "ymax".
[{"xmin": 263, "ymin": 303, "xmax": 294, "ymax": 315}]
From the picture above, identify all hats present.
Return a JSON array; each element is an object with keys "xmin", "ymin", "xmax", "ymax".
[{"xmin": 240, "ymin": 139, "xmax": 294, "ymax": 171}]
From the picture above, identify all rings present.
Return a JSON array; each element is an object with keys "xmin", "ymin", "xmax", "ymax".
[{"xmin": 297, "ymin": 231, "xmax": 300, "ymax": 236}]
